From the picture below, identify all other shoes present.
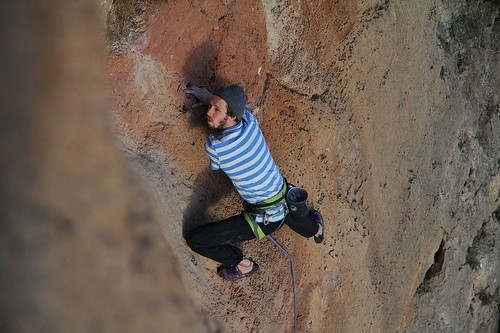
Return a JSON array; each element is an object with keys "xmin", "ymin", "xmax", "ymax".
[
  {"xmin": 217, "ymin": 257, "xmax": 259, "ymax": 281},
  {"xmin": 309, "ymin": 209, "xmax": 324, "ymax": 243}
]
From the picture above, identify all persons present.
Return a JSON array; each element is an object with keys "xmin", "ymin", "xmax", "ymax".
[{"xmin": 182, "ymin": 83, "xmax": 326, "ymax": 283}]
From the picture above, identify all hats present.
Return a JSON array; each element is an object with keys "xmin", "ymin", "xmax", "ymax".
[{"xmin": 214, "ymin": 85, "xmax": 248, "ymax": 121}]
